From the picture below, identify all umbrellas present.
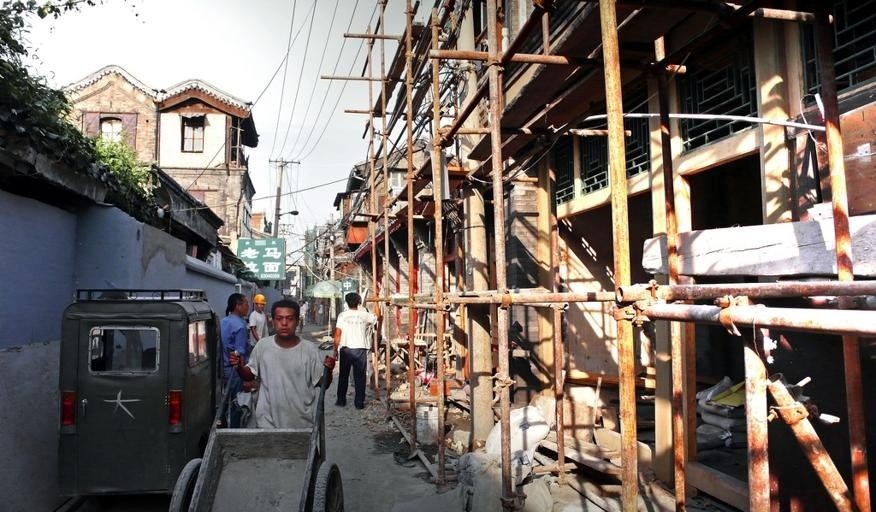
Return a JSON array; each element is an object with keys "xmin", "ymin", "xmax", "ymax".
[{"xmin": 301, "ymin": 278, "xmax": 342, "ymax": 340}]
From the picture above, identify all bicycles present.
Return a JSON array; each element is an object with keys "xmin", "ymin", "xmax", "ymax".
[{"xmin": 299, "ymin": 313, "xmax": 304, "ymax": 331}]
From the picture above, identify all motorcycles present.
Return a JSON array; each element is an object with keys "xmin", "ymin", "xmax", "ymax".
[{"xmin": 51, "ymin": 281, "xmax": 224, "ymax": 506}]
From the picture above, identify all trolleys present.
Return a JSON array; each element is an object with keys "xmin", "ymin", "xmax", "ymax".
[{"xmin": 163, "ymin": 353, "xmax": 345, "ymax": 512}]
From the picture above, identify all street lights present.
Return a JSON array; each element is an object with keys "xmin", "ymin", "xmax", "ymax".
[{"xmin": 269, "ymin": 209, "xmax": 299, "ymax": 287}]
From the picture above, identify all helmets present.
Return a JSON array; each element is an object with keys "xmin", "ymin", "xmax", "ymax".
[{"xmin": 252, "ymin": 294, "xmax": 267, "ymax": 307}]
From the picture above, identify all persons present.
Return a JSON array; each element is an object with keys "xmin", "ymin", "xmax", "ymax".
[
  {"xmin": 296, "ymin": 297, "xmax": 325, "ymax": 333},
  {"xmin": 332, "ymin": 292, "xmax": 378, "ymax": 409},
  {"xmin": 248, "ymin": 294, "xmax": 269, "ymax": 357},
  {"xmin": 228, "ymin": 298, "xmax": 335, "ymax": 431},
  {"xmin": 218, "ymin": 292, "xmax": 251, "ymax": 428}
]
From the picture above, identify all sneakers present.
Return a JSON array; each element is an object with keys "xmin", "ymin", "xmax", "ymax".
[
  {"xmin": 334, "ymin": 401, "xmax": 346, "ymax": 406},
  {"xmin": 353, "ymin": 401, "xmax": 364, "ymax": 409}
]
